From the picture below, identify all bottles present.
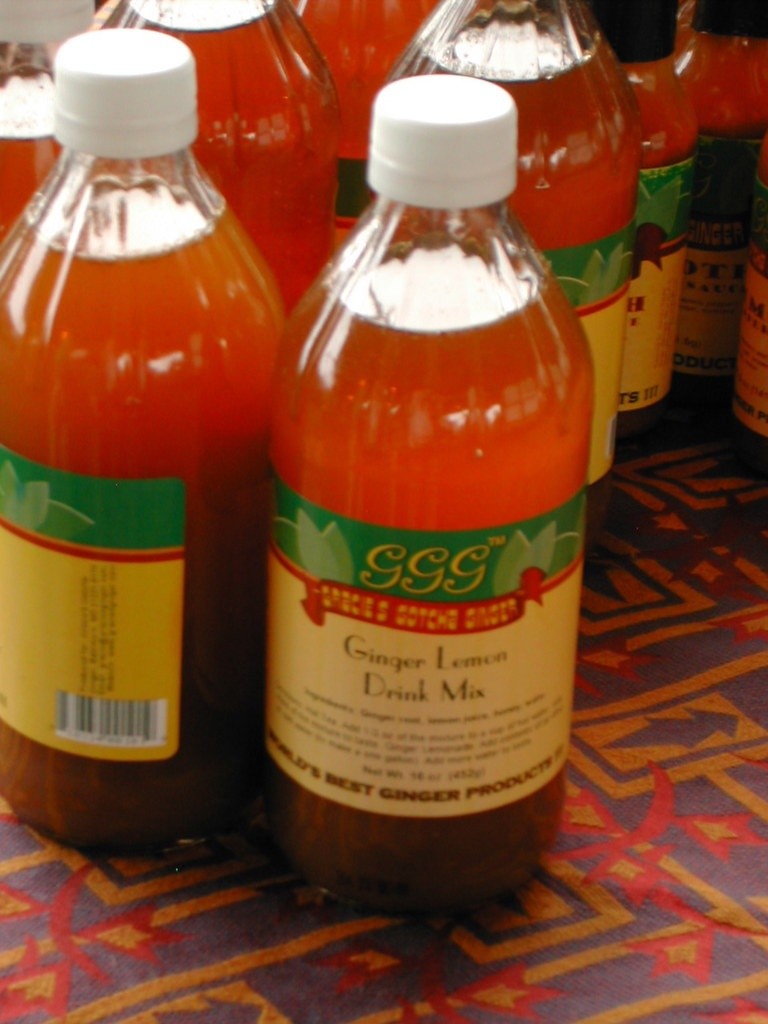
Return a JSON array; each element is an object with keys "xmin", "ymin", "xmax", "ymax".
[
  {"xmin": 284, "ymin": 1, "xmax": 767, "ymax": 550},
  {"xmin": 255, "ymin": 72, "xmax": 596, "ymax": 915},
  {"xmin": 68, "ymin": 3, "xmax": 345, "ymax": 328},
  {"xmin": 1, "ymin": 31, "xmax": 290, "ymax": 852}
]
